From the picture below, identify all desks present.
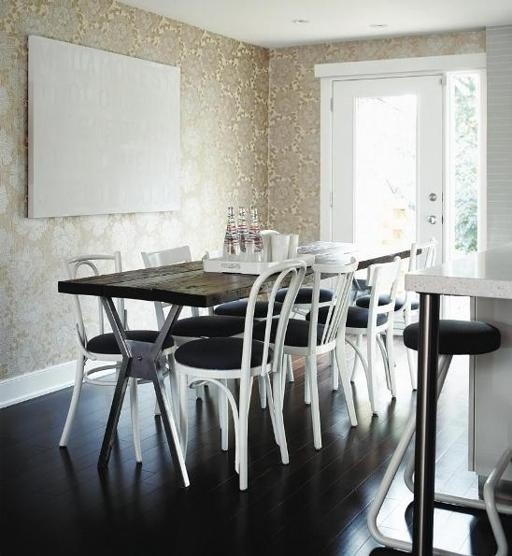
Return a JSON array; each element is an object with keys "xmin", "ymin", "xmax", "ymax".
[
  {"xmin": 404, "ymin": 241, "xmax": 511, "ymax": 515},
  {"xmin": 58, "ymin": 245, "xmax": 422, "ymax": 486}
]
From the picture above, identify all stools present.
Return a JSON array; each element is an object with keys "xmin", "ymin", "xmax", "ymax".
[{"xmin": 368, "ymin": 317, "xmax": 512, "ymax": 556}]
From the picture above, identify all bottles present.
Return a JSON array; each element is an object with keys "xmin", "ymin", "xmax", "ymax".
[{"xmin": 222, "ymin": 206, "xmax": 264, "ymax": 262}]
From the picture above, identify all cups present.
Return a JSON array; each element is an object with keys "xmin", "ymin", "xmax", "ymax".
[{"xmin": 261, "ymin": 230, "xmax": 299, "ymax": 263}]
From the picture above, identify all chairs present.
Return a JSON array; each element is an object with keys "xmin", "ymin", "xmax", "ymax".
[
  {"xmin": 263, "ymin": 229, "xmax": 340, "ymax": 383},
  {"xmin": 141, "ymin": 246, "xmax": 267, "ymax": 410},
  {"xmin": 58, "ymin": 251, "xmax": 179, "ymax": 463},
  {"xmin": 304, "ymin": 254, "xmax": 403, "ymax": 418},
  {"xmin": 221, "ymin": 255, "xmax": 360, "ymax": 451},
  {"xmin": 174, "ymin": 259, "xmax": 307, "ymax": 491},
  {"xmin": 332, "ymin": 238, "xmax": 437, "ymax": 391}
]
